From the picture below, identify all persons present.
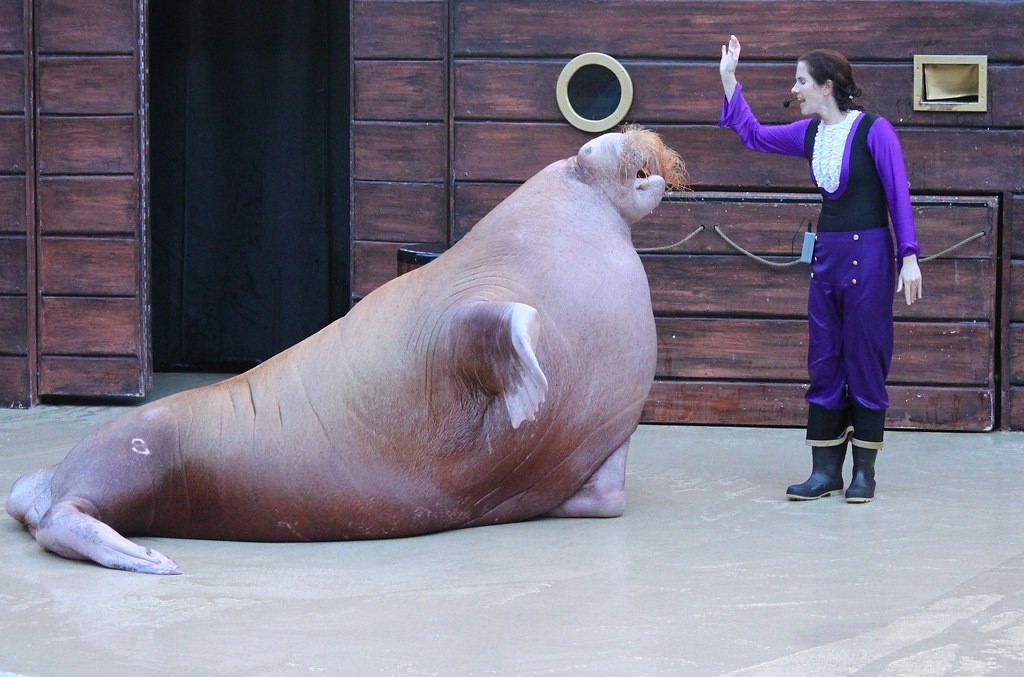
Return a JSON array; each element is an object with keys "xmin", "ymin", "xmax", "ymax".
[{"xmin": 716, "ymin": 33, "xmax": 922, "ymax": 504}]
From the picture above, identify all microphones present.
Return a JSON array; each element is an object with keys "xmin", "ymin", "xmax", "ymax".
[{"xmin": 783, "ymin": 97, "xmax": 797, "ymax": 107}]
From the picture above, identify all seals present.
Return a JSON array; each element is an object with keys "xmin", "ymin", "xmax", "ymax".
[{"xmin": 6, "ymin": 126, "xmax": 689, "ymax": 577}]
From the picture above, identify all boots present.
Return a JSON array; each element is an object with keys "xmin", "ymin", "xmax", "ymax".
[
  {"xmin": 845, "ymin": 444, "xmax": 877, "ymax": 503},
  {"xmin": 786, "ymin": 439, "xmax": 849, "ymax": 500}
]
